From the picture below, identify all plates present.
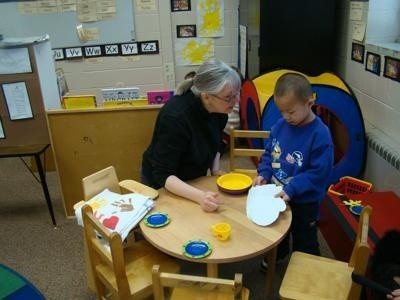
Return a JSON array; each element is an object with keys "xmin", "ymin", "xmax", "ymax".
[
  {"xmin": 144, "ymin": 211, "xmax": 170, "ymax": 228},
  {"xmin": 181, "ymin": 238, "xmax": 212, "ymax": 260},
  {"xmin": 216, "ymin": 173, "xmax": 252, "ymax": 195}
]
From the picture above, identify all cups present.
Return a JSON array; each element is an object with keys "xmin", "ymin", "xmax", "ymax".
[{"xmin": 211, "ymin": 222, "xmax": 231, "ymax": 241}]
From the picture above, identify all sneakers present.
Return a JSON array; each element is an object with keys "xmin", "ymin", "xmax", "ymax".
[{"xmin": 260, "ymin": 257, "xmax": 271, "ymax": 272}]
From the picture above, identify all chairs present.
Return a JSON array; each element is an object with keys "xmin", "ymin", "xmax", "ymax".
[
  {"xmin": 151, "ymin": 264, "xmax": 251, "ymax": 300},
  {"xmin": 278, "ymin": 205, "xmax": 373, "ymax": 300},
  {"xmin": 230, "ymin": 126, "xmax": 270, "ymax": 176},
  {"xmin": 73, "ymin": 166, "xmax": 159, "ymax": 248},
  {"xmin": 81, "ymin": 204, "xmax": 181, "ymax": 300}
]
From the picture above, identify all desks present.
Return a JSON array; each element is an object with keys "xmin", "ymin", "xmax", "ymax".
[
  {"xmin": 139, "ymin": 176, "xmax": 293, "ymax": 300},
  {"xmin": 45, "ymin": 99, "xmax": 166, "ymax": 219}
]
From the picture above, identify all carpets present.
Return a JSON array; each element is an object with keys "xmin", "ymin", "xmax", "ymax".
[{"xmin": 0, "ymin": 262, "xmax": 46, "ymax": 300}]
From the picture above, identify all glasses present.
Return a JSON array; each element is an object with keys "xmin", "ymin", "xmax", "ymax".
[{"xmin": 208, "ymin": 93, "xmax": 238, "ymax": 102}]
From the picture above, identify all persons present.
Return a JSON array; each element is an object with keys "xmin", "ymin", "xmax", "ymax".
[
  {"xmin": 255, "ymin": 72, "xmax": 334, "ymax": 265},
  {"xmin": 141, "ymin": 60, "xmax": 249, "ymax": 212}
]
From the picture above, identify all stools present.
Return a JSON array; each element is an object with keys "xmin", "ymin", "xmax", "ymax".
[{"xmin": 0, "ymin": 143, "xmax": 57, "ymax": 226}]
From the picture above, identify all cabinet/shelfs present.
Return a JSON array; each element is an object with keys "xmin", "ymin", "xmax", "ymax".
[{"xmin": 0, "ymin": 38, "xmax": 63, "ymax": 172}]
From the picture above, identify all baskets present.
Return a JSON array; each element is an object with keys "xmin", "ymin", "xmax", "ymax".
[{"xmin": 328, "ymin": 176, "xmax": 372, "ymax": 197}]
